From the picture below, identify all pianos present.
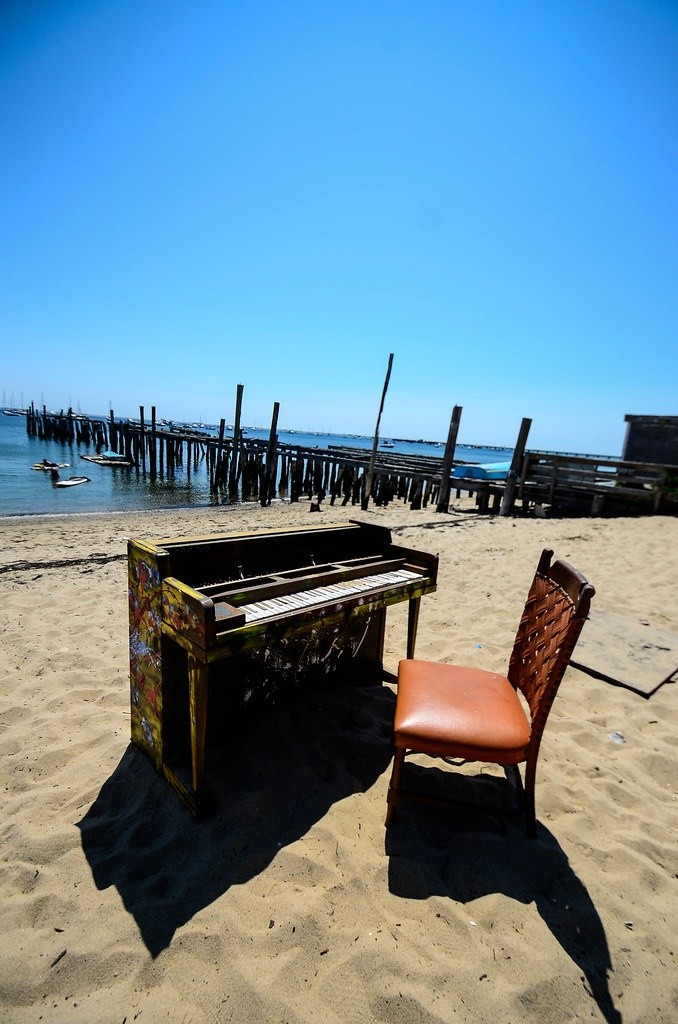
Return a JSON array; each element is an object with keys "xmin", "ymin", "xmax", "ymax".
[{"xmin": 127, "ymin": 518, "xmax": 441, "ymax": 826}]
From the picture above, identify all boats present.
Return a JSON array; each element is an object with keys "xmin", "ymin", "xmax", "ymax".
[
  {"xmin": 30, "ymin": 459, "xmax": 69, "ymax": 470},
  {"xmin": 55, "ymin": 476, "xmax": 91, "ymax": 486},
  {"xmin": 434, "ymin": 445, "xmax": 439, "ymax": 448},
  {"xmin": 380, "ymin": 441, "xmax": 395, "ymax": 448},
  {"xmin": 468, "ymin": 447, "xmax": 471, "ymax": 450},
  {"xmin": 80, "ymin": 450, "xmax": 130, "ymax": 467},
  {"xmin": 155, "ymin": 418, "xmax": 324, "ymax": 438},
  {"xmin": 106, "ymin": 400, "xmax": 112, "ymax": 421}
]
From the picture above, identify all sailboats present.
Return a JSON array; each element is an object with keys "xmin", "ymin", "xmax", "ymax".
[{"xmin": 2, "ymin": 389, "xmax": 85, "ymax": 419}]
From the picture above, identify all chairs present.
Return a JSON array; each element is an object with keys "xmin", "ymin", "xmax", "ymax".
[{"xmin": 386, "ymin": 549, "xmax": 595, "ymax": 839}]
